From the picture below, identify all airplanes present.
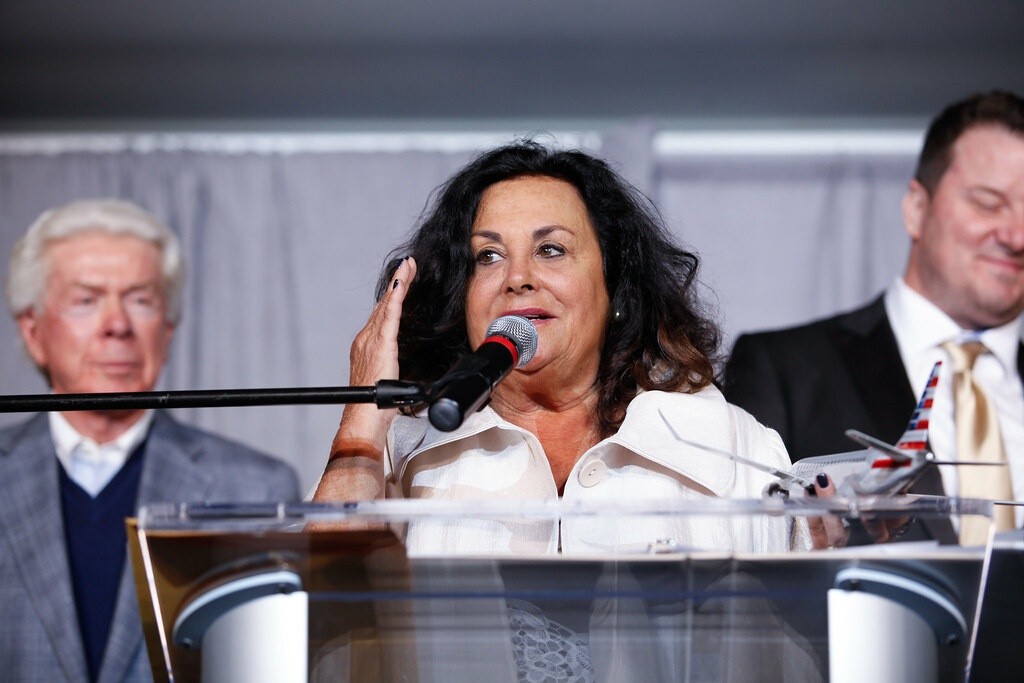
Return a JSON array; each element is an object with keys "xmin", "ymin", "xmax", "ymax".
[{"xmin": 656, "ymin": 360, "xmax": 1024, "ymax": 523}]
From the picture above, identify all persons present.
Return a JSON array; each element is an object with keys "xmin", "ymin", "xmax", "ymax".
[
  {"xmin": 0, "ymin": 198, "xmax": 301, "ymax": 683},
  {"xmin": 724, "ymin": 90, "xmax": 1024, "ymax": 549},
  {"xmin": 305, "ymin": 140, "xmax": 913, "ymax": 556}
]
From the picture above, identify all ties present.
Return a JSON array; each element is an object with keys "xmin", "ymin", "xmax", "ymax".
[{"xmin": 945, "ymin": 343, "xmax": 1014, "ymax": 544}]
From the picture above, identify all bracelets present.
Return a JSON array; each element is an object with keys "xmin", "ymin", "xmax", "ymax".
[{"xmin": 328, "ymin": 446, "xmax": 384, "ymax": 461}]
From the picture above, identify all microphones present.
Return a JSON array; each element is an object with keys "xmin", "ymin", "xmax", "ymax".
[{"xmin": 428, "ymin": 315, "xmax": 539, "ymax": 433}]
